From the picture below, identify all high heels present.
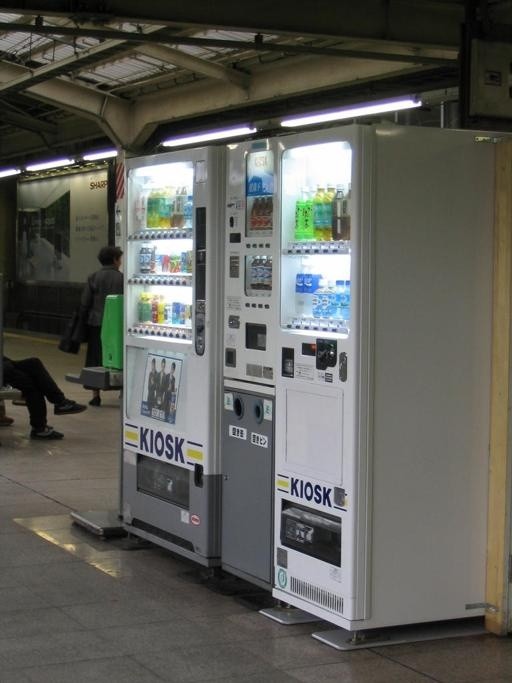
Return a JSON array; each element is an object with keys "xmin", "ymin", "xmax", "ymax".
[{"xmin": 89, "ymin": 397, "xmax": 101, "ymax": 407}]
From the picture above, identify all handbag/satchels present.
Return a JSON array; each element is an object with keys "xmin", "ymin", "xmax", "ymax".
[{"xmin": 58, "ymin": 307, "xmax": 89, "ymax": 354}]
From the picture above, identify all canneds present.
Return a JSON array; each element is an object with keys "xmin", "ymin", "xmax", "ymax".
[
  {"xmin": 186, "ymin": 251, "xmax": 192, "ymax": 273},
  {"xmin": 181, "ymin": 252, "xmax": 186, "ymax": 273},
  {"xmin": 172, "ymin": 302, "xmax": 192, "ymax": 327}
]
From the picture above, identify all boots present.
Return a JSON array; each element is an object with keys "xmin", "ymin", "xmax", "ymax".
[{"xmin": 0, "ymin": 406, "xmax": 14, "ymax": 426}]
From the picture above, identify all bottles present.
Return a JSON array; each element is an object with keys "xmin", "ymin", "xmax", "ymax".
[
  {"xmin": 138, "ymin": 184, "xmax": 193, "ymax": 228},
  {"xmin": 139, "ymin": 243, "xmax": 192, "ymax": 273},
  {"xmin": 295, "ymin": 181, "xmax": 351, "ymax": 240},
  {"xmin": 250, "ymin": 256, "xmax": 272, "ymax": 290},
  {"xmin": 295, "ymin": 265, "xmax": 350, "ymax": 319},
  {"xmin": 250, "ymin": 196, "xmax": 273, "ymax": 230},
  {"xmin": 139, "ymin": 292, "xmax": 192, "ymax": 324}
]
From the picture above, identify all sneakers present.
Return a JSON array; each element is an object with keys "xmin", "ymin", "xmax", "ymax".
[
  {"xmin": 54, "ymin": 399, "xmax": 87, "ymax": 415},
  {"xmin": 31, "ymin": 425, "xmax": 65, "ymax": 440}
]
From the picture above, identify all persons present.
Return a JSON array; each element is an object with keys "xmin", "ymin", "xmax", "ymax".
[
  {"xmin": 0, "ymin": 355, "xmax": 87, "ymax": 439},
  {"xmin": 80, "ymin": 246, "xmax": 123, "ymax": 406},
  {"xmin": 148, "ymin": 358, "xmax": 175, "ymax": 419}
]
